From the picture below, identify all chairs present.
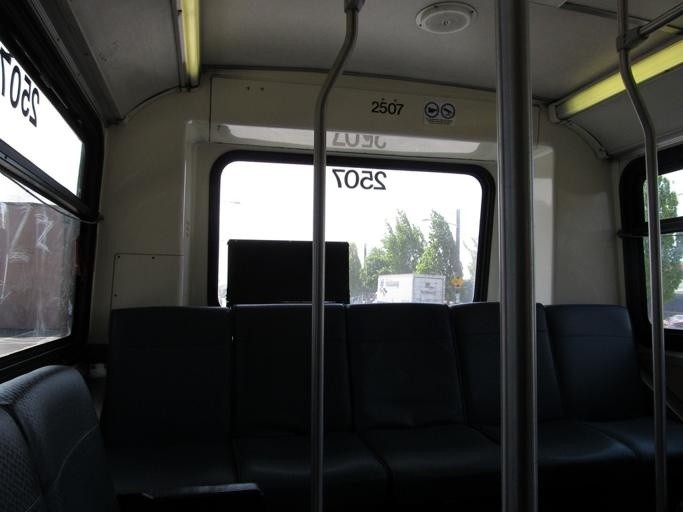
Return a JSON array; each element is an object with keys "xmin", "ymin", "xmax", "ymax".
[{"xmin": 1, "ymin": 301, "xmax": 682, "ymax": 511}]
